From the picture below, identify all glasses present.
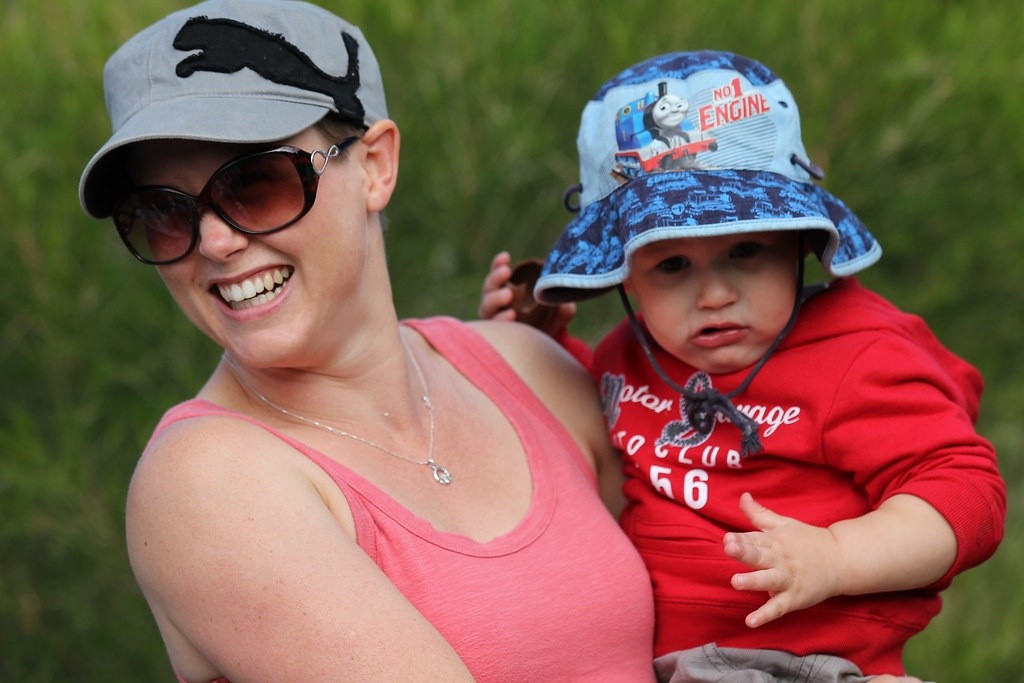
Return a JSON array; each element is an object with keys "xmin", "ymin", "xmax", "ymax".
[{"xmin": 108, "ymin": 143, "xmax": 341, "ymax": 268}]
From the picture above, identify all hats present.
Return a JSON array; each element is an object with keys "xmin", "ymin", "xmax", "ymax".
[
  {"xmin": 78, "ymin": 0, "xmax": 390, "ymax": 220},
  {"xmin": 531, "ymin": 48, "xmax": 883, "ymax": 307}
]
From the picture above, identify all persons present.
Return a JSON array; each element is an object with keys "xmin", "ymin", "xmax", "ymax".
[
  {"xmin": 77, "ymin": 0, "xmax": 656, "ymax": 683},
  {"xmin": 479, "ymin": 51, "xmax": 1008, "ymax": 683}
]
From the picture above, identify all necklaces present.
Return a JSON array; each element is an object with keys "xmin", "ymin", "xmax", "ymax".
[{"xmin": 220, "ymin": 322, "xmax": 452, "ymax": 485}]
